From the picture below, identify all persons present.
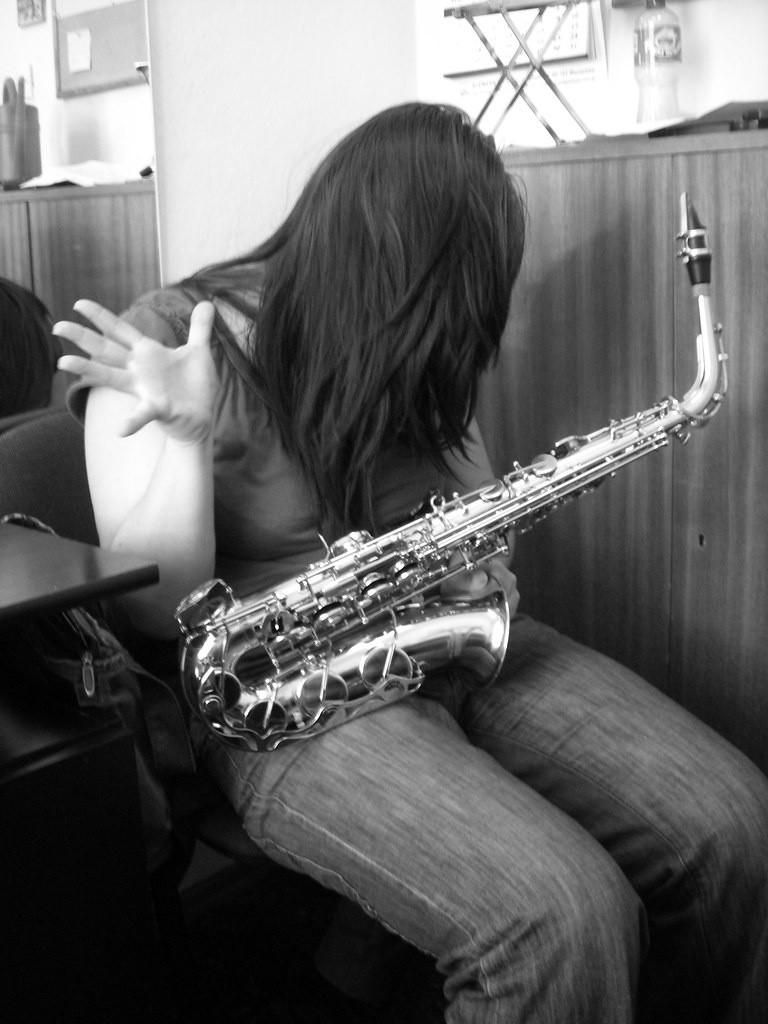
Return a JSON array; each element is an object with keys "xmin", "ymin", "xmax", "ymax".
[{"xmin": 52, "ymin": 102, "xmax": 768, "ymax": 1024}]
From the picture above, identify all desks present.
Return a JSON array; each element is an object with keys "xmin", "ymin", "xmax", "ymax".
[{"xmin": 0, "ymin": 520, "xmax": 165, "ymax": 1024}]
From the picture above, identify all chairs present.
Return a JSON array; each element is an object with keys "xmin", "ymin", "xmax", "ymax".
[{"xmin": 0, "ymin": 406, "xmax": 270, "ymax": 1024}]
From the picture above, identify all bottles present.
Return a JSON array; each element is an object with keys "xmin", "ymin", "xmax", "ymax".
[{"xmin": 634, "ymin": 0, "xmax": 684, "ymax": 125}]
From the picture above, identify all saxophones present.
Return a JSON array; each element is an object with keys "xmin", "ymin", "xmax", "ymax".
[{"xmin": 174, "ymin": 194, "xmax": 729, "ymax": 754}]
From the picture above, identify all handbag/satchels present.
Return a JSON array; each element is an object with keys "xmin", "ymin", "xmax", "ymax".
[{"xmin": 0, "ymin": 513, "xmax": 197, "ymax": 907}]
